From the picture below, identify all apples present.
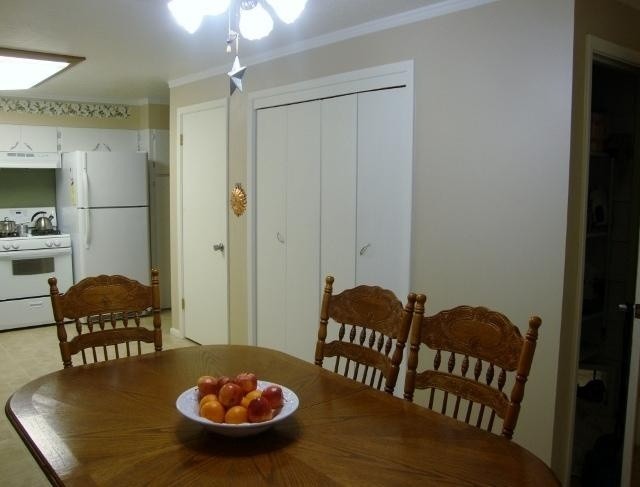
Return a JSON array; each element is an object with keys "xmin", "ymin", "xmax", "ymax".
[{"xmin": 197, "ymin": 372, "xmax": 283, "ymax": 424}]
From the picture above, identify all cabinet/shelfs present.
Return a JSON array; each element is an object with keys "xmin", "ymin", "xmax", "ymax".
[
  {"xmin": 0, "ymin": 125, "xmax": 60, "ymax": 153},
  {"xmin": 58, "ymin": 126, "xmax": 141, "ymax": 155},
  {"xmin": 149, "ymin": 130, "xmax": 171, "ymax": 310}
]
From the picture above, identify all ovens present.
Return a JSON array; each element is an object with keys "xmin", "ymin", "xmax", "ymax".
[{"xmin": 1, "ymin": 247, "xmax": 77, "ymax": 332}]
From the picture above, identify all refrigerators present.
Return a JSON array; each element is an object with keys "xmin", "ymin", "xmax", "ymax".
[{"xmin": 55, "ymin": 150, "xmax": 154, "ymax": 324}]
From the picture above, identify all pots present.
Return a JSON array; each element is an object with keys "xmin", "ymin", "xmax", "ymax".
[{"xmin": 0, "ymin": 217, "xmax": 16, "ymax": 235}]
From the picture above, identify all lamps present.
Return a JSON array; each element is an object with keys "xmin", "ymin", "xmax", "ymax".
[
  {"xmin": 1, "ymin": 47, "xmax": 86, "ymax": 91},
  {"xmin": 167, "ymin": 2, "xmax": 305, "ymax": 90}
]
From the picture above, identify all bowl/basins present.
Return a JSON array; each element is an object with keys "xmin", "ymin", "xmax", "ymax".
[{"xmin": 176, "ymin": 378, "xmax": 301, "ymax": 437}]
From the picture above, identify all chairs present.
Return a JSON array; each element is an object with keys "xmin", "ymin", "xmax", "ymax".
[
  {"xmin": 48, "ymin": 269, "xmax": 161, "ymax": 368},
  {"xmin": 314, "ymin": 275, "xmax": 414, "ymax": 397},
  {"xmin": 404, "ymin": 292, "xmax": 543, "ymax": 440}
]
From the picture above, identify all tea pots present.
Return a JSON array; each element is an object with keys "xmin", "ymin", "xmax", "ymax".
[{"xmin": 31, "ymin": 211, "xmax": 54, "ymax": 232}]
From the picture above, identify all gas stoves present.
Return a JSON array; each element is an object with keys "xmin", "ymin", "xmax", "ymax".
[{"xmin": 0, "ymin": 230, "xmax": 61, "ymax": 237}]
